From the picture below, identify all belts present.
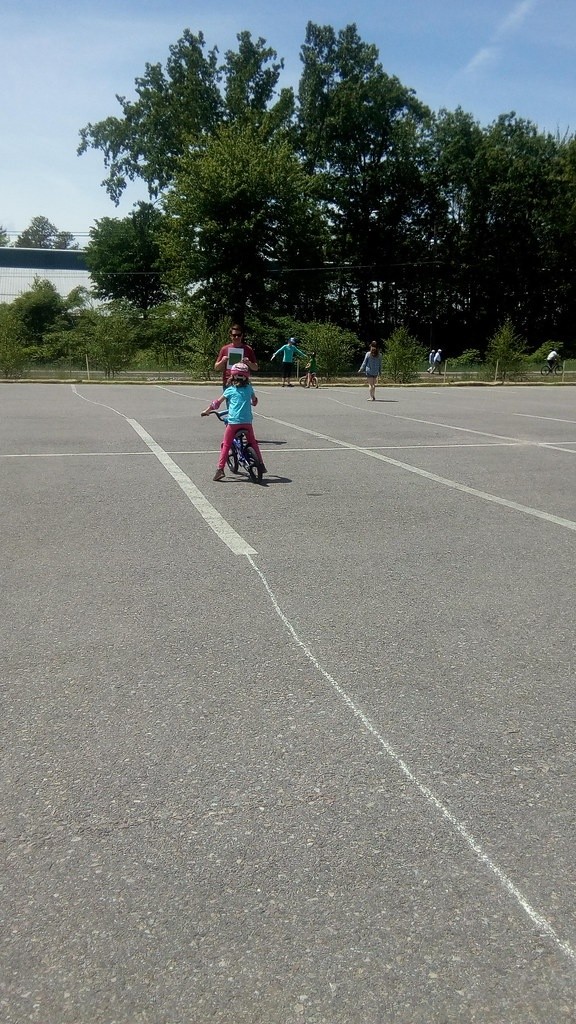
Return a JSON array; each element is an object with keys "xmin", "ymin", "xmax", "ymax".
[{"xmin": 437, "ymin": 360, "xmax": 439, "ymax": 363}]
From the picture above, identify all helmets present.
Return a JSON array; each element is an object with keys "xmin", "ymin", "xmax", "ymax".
[{"xmin": 231, "ymin": 363, "xmax": 249, "ymax": 377}]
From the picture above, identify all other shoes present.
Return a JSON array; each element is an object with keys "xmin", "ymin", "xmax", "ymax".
[
  {"xmin": 430, "ymin": 372, "xmax": 434, "ymax": 374},
  {"xmin": 367, "ymin": 397, "xmax": 374, "ymax": 401},
  {"xmin": 306, "ymin": 386, "xmax": 309, "ymax": 388},
  {"xmin": 282, "ymin": 384, "xmax": 284, "ymax": 387},
  {"xmin": 287, "ymin": 384, "xmax": 294, "ymax": 387},
  {"xmin": 261, "ymin": 463, "xmax": 268, "ymax": 473},
  {"xmin": 213, "ymin": 469, "xmax": 225, "ymax": 481},
  {"xmin": 427, "ymin": 370, "xmax": 430, "ymax": 373},
  {"xmin": 439, "ymin": 374, "xmax": 443, "ymax": 375}
]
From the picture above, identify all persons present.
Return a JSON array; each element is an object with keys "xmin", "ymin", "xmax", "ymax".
[
  {"xmin": 202, "ymin": 363, "xmax": 268, "ymax": 481},
  {"xmin": 215, "ymin": 325, "xmax": 258, "ymax": 409},
  {"xmin": 305, "ymin": 352, "xmax": 316, "ymax": 388},
  {"xmin": 271, "ymin": 337, "xmax": 309, "ymax": 388},
  {"xmin": 430, "ymin": 349, "xmax": 443, "ymax": 375},
  {"xmin": 357, "ymin": 341, "xmax": 382, "ymax": 401},
  {"xmin": 427, "ymin": 350, "xmax": 435, "ymax": 372},
  {"xmin": 546, "ymin": 348, "xmax": 561, "ymax": 373}
]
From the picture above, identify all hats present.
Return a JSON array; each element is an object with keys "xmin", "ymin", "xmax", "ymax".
[
  {"xmin": 554, "ymin": 348, "xmax": 558, "ymax": 350},
  {"xmin": 438, "ymin": 349, "xmax": 442, "ymax": 352},
  {"xmin": 431, "ymin": 350, "xmax": 435, "ymax": 352},
  {"xmin": 289, "ymin": 338, "xmax": 295, "ymax": 344}
]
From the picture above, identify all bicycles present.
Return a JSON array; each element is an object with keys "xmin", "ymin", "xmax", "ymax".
[
  {"xmin": 541, "ymin": 361, "xmax": 563, "ymax": 376},
  {"xmin": 299, "ymin": 368, "xmax": 318, "ymax": 389},
  {"xmin": 201, "ymin": 403, "xmax": 263, "ymax": 484}
]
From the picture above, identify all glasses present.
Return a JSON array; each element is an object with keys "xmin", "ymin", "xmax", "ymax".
[{"xmin": 231, "ymin": 332, "xmax": 242, "ymax": 337}]
